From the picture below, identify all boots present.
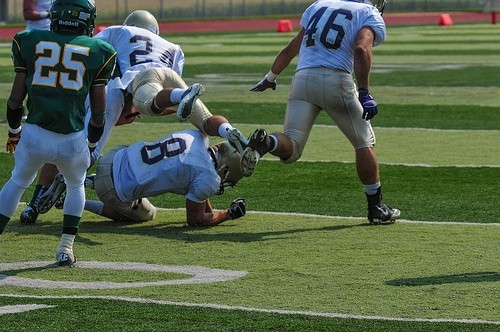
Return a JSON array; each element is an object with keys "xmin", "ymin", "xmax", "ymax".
[
  {"xmin": 240, "ymin": 128, "xmax": 275, "ymax": 177},
  {"xmin": 20, "ymin": 183, "xmax": 49, "ymax": 223},
  {"xmin": 366, "ymin": 186, "xmax": 401, "ymax": 223}
]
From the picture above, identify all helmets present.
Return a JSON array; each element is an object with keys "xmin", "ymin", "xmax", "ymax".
[
  {"xmin": 124, "ymin": 10, "xmax": 159, "ymax": 35},
  {"xmin": 23, "ymin": 0, "xmax": 55, "ymax": 21},
  {"xmin": 210, "ymin": 141, "xmax": 244, "ymax": 187},
  {"xmin": 48, "ymin": 0, "xmax": 96, "ymax": 36},
  {"xmin": 370, "ymin": 0, "xmax": 388, "ymax": 15}
]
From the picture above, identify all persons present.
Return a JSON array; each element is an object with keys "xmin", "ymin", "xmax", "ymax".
[
  {"xmin": 21, "ymin": 0, "xmax": 261, "ymax": 227},
  {"xmin": 0, "ymin": 0, "xmax": 118, "ymax": 265},
  {"xmin": 239, "ymin": 0, "xmax": 402, "ymax": 224}
]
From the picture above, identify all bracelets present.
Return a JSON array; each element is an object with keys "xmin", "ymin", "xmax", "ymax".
[{"xmin": 358, "ymin": 88, "xmax": 369, "ymax": 93}]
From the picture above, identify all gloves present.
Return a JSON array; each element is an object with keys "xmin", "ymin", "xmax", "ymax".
[
  {"xmin": 250, "ymin": 71, "xmax": 276, "ymax": 92},
  {"xmin": 5, "ymin": 129, "xmax": 22, "ymax": 153},
  {"xmin": 228, "ymin": 196, "xmax": 246, "ymax": 220},
  {"xmin": 359, "ymin": 91, "xmax": 378, "ymax": 121}
]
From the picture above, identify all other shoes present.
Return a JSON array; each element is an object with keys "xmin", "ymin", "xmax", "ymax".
[
  {"xmin": 56, "ymin": 253, "xmax": 78, "ymax": 267},
  {"xmin": 53, "ymin": 192, "xmax": 66, "ymax": 210},
  {"xmin": 227, "ymin": 128, "xmax": 250, "ymax": 155},
  {"xmin": 37, "ymin": 174, "xmax": 66, "ymax": 214},
  {"xmin": 177, "ymin": 84, "xmax": 204, "ymax": 122}
]
